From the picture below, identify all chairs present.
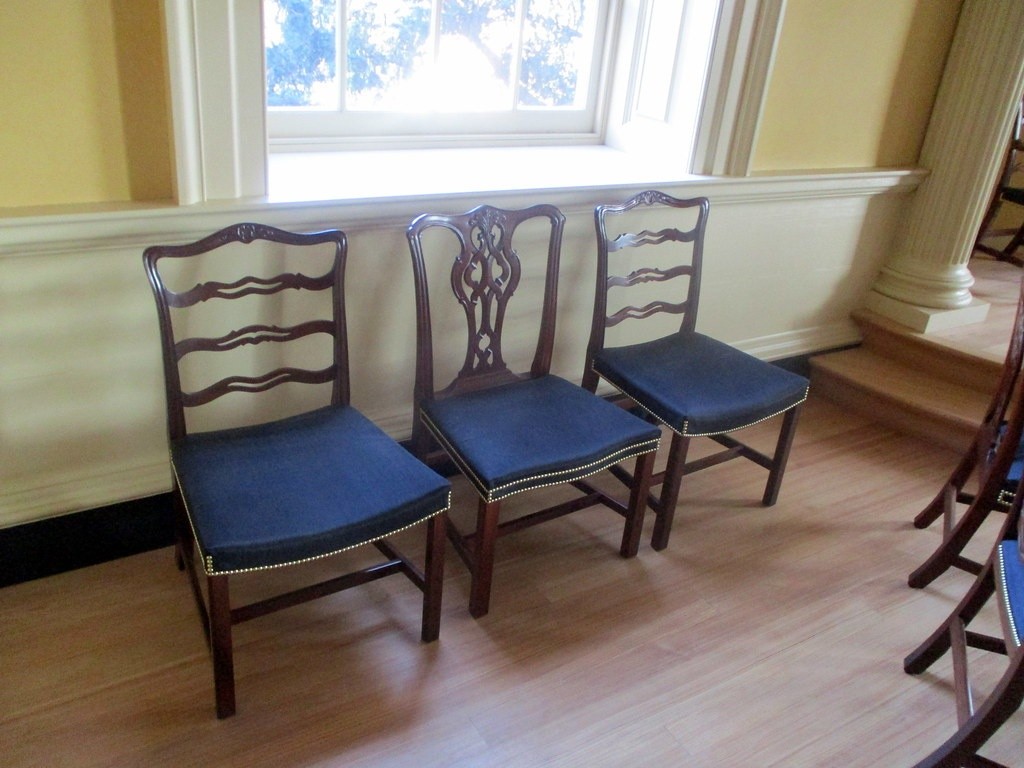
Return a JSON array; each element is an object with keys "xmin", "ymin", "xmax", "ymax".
[
  {"xmin": 142, "ymin": 222, "xmax": 453, "ymax": 719},
  {"xmin": 405, "ymin": 205, "xmax": 662, "ymax": 616},
  {"xmin": 903, "ymin": 468, "xmax": 1023, "ymax": 768},
  {"xmin": 908, "ymin": 274, "xmax": 1024, "ymax": 589},
  {"xmin": 581, "ymin": 190, "xmax": 810, "ymax": 552}
]
